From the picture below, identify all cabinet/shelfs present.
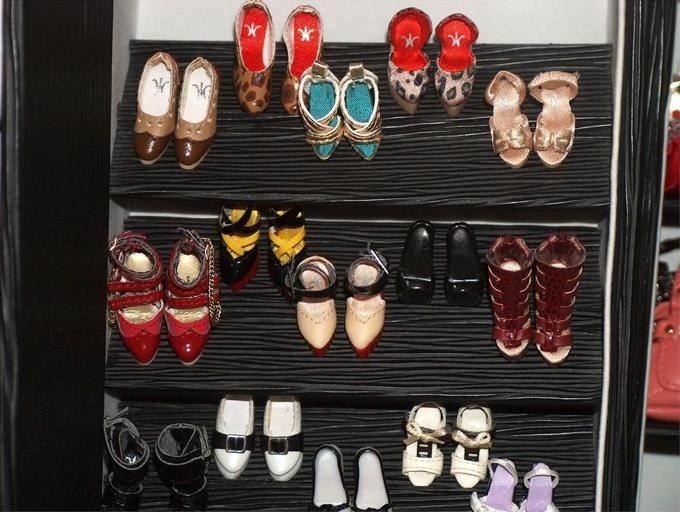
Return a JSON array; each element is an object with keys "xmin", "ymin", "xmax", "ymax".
[{"xmin": 2, "ymin": 2, "xmax": 678, "ymax": 511}]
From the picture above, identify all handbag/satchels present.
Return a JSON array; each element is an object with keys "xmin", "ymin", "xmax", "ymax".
[{"xmin": 647, "ymin": 301, "xmax": 680, "ymax": 422}]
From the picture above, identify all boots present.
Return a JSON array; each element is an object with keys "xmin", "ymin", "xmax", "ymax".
[{"xmin": 103, "ymin": 407, "xmax": 213, "ymax": 512}]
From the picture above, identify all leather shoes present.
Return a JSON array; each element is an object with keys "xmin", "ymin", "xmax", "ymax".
[
  {"xmin": 312, "ymin": 444, "xmax": 393, "ymax": 512},
  {"xmin": 281, "ymin": 6, "xmax": 323, "ymax": 115},
  {"xmin": 217, "ymin": 205, "xmax": 261, "ymax": 291},
  {"xmin": 285, "ymin": 256, "xmax": 339, "ymax": 357},
  {"xmin": 434, "ymin": 13, "xmax": 479, "ymax": 117},
  {"xmin": 262, "ymin": 396, "xmax": 304, "ymax": 481},
  {"xmin": 445, "ymin": 222, "xmax": 483, "ymax": 307},
  {"xmin": 234, "ymin": 0, "xmax": 276, "ymax": 114},
  {"xmin": 345, "ymin": 250, "xmax": 391, "ymax": 359},
  {"xmin": 396, "ymin": 221, "xmax": 434, "ymax": 305},
  {"xmin": 134, "ymin": 51, "xmax": 220, "ymax": 170},
  {"xmin": 268, "ymin": 207, "xmax": 305, "ymax": 305},
  {"xmin": 107, "ymin": 228, "xmax": 223, "ymax": 366},
  {"xmin": 388, "ymin": 8, "xmax": 432, "ymax": 115},
  {"xmin": 213, "ymin": 393, "xmax": 254, "ymax": 480}
]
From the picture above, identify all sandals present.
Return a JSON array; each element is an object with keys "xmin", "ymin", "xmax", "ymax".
[
  {"xmin": 450, "ymin": 404, "xmax": 496, "ymax": 489},
  {"xmin": 485, "ymin": 70, "xmax": 580, "ymax": 168},
  {"xmin": 401, "ymin": 401, "xmax": 446, "ymax": 486},
  {"xmin": 298, "ymin": 61, "xmax": 383, "ymax": 161},
  {"xmin": 470, "ymin": 458, "xmax": 560, "ymax": 512},
  {"xmin": 486, "ymin": 235, "xmax": 586, "ymax": 364}
]
